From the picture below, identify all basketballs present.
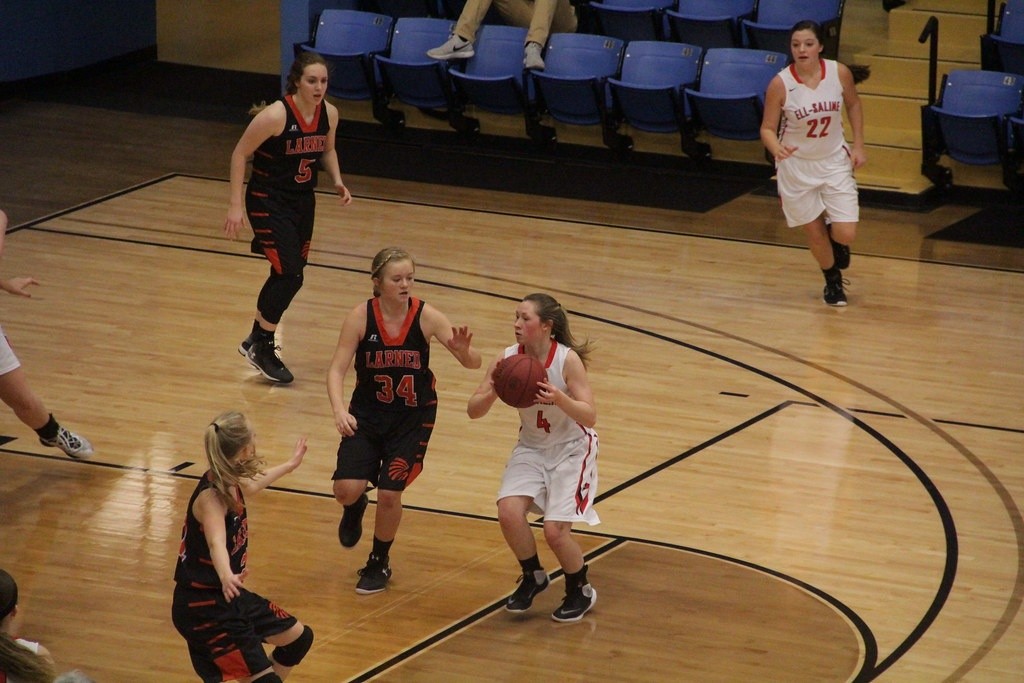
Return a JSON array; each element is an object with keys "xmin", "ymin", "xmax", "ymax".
[{"xmin": 492, "ymin": 354, "xmax": 548, "ymax": 408}]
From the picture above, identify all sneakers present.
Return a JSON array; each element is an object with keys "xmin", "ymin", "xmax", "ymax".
[
  {"xmin": 355, "ymin": 552, "xmax": 392, "ymax": 594},
  {"xmin": 505, "ymin": 567, "xmax": 549, "ymax": 613},
  {"xmin": 426, "ymin": 35, "xmax": 475, "ymax": 59},
  {"xmin": 338, "ymin": 494, "xmax": 368, "ymax": 548},
  {"xmin": 829, "ymin": 226, "xmax": 851, "ymax": 269},
  {"xmin": 40, "ymin": 425, "xmax": 93, "ymax": 460},
  {"xmin": 551, "ymin": 582, "xmax": 596, "ymax": 623},
  {"xmin": 823, "ymin": 277, "xmax": 852, "ymax": 307},
  {"xmin": 523, "ymin": 43, "xmax": 545, "ymax": 71},
  {"xmin": 238, "ymin": 334, "xmax": 294, "ymax": 383}
]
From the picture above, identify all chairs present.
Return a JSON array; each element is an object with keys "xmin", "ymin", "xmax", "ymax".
[
  {"xmin": 294, "ymin": 6, "xmax": 396, "ymax": 126},
  {"xmin": 740, "ymin": 0, "xmax": 846, "ymax": 60},
  {"xmin": 1005, "ymin": 99, "xmax": 1024, "ymax": 192},
  {"xmin": 604, "ymin": 39, "xmax": 707, "ymax": 163},
  {"xmin": 523, "ymin": 31, "xmax": 627, "ymax": 152},
  {"xmin": 447, "ymin": 24, "xmax": 542, "ymax": 144},
  {"xmin": 986, "ymin": 0, "xmax": 1024, "ymax": 72},
  {"xmin": 681, "ymin": 45, "xmax": 790, "ymax": 169},
  {"xmin": 926, "ymin": 67, "xmax": 1024, "ymax": 188},
  {"xmin": 589, "ymin": 0, "xmax": 675, "ymax": 42},
  {"xmin": 373, "ymin": 13, "xmax": 468, "ymax": 139},
  {"xmin": 665, "ymin": 0, "xmax": 754, "ymax": 48}
]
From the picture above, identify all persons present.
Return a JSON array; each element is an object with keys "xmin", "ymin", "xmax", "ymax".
[
  {"xmin": 1, "ymin": 210, "xmax": 92, "ymax": 459},
  {"xmin": 328, "ymin": 247, "xmax": 481, "ymax": 594},
  {"xmin": 759, "ymin": 19, "xmax": 865, "ymax": 305},
  {"xmin": 467, "ymin": 293, "xmax": 601, "ymax": 621},
  {"xmin": 426, "ymin": 0, "xmax": 597, "ymax": 70},
  {"xmin": 172, "ymin": 410, "xmax": 314, "ymax": 683},
  {"xmin": 0, "ymin": 568, "xmax": 57, "ymax": 683},
  {"xmin": 225, "ymin": 53, "xmax": 351, "ymax": 382}
]
